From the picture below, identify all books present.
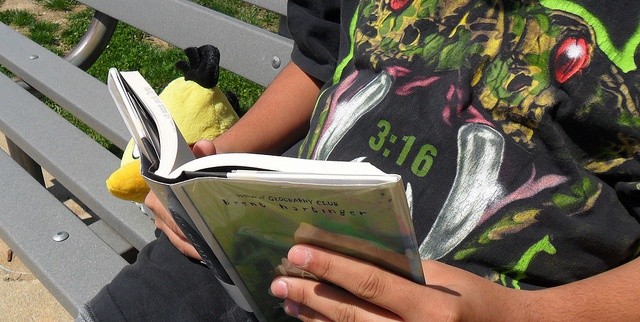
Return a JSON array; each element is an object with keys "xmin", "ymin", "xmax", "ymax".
[{"xmin": 107, "ymin": 66, "xmax": 426, "ymax": 322}]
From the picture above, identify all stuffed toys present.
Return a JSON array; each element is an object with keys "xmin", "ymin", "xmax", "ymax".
[{"xmin": 106, "ymin": 44, "xmax": 241, "ymax": 223}]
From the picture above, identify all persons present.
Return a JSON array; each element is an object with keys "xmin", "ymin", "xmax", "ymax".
[{"xmin": 78, "ymin": 0, "xmax": 640, "ymax": 322}]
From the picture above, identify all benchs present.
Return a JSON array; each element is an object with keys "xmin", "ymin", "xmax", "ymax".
[{"xmin": 0, "ymin": 0, "xmax": 300, "ymax": 321}]
